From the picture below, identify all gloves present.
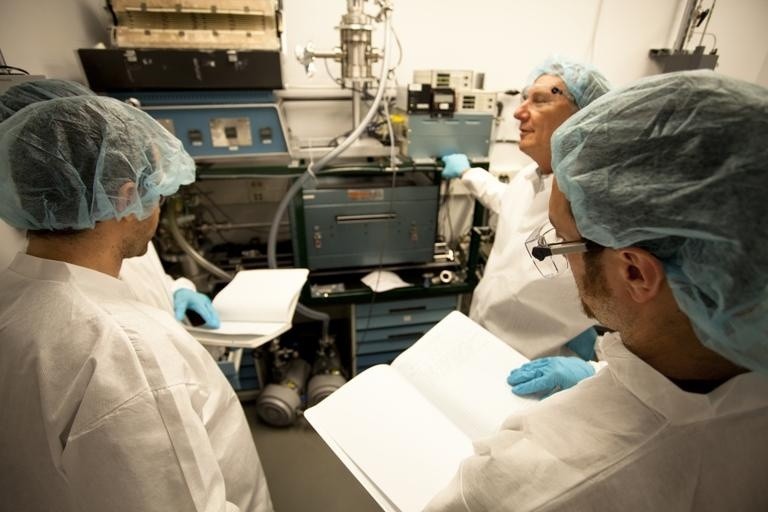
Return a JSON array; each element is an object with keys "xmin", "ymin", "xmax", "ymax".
[
  {"xmin": 440, "ymin": 151, "xmax": 471, "ymax": 178},
  {"xmin": 507, "ymin": 352, "xmax": 594, "ymax": 397},
  {"xmin": 173, "ymin": 287, "xmax": 221, "ymax": 329}
]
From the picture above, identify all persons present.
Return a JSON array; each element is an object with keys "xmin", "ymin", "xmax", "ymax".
[
  {"xmin": 439, "ymin": 62, "xmax": 612, "ymax": 364},
  {"xmin": 0, "ymin": 80, "xmax": 219, "ymax": 332},
  {"xmin": 0, "ymin": 98, "xmax": 273, "ymax": 512},
  {"xmin": 422, "ymin": 70, "xmax": 768, "ymax": 512}
]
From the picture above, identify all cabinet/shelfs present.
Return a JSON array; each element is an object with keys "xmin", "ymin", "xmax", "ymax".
[{"xmin": 349, "ymin": 294, "xmax": 462, "ymax": 379}]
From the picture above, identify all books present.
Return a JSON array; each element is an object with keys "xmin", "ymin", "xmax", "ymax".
[
  {"xmin": 186, "ymin": 268, "xmax": 310, "ymax": 350},
  {"xmin": 305, "ymin": 310, "xmax": 558, "ymax": 512}
]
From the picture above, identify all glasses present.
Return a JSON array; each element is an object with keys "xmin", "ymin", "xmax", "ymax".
[{"xmin": 524, "ymin": 219, "xmax": 687, "ymax": 279}]
